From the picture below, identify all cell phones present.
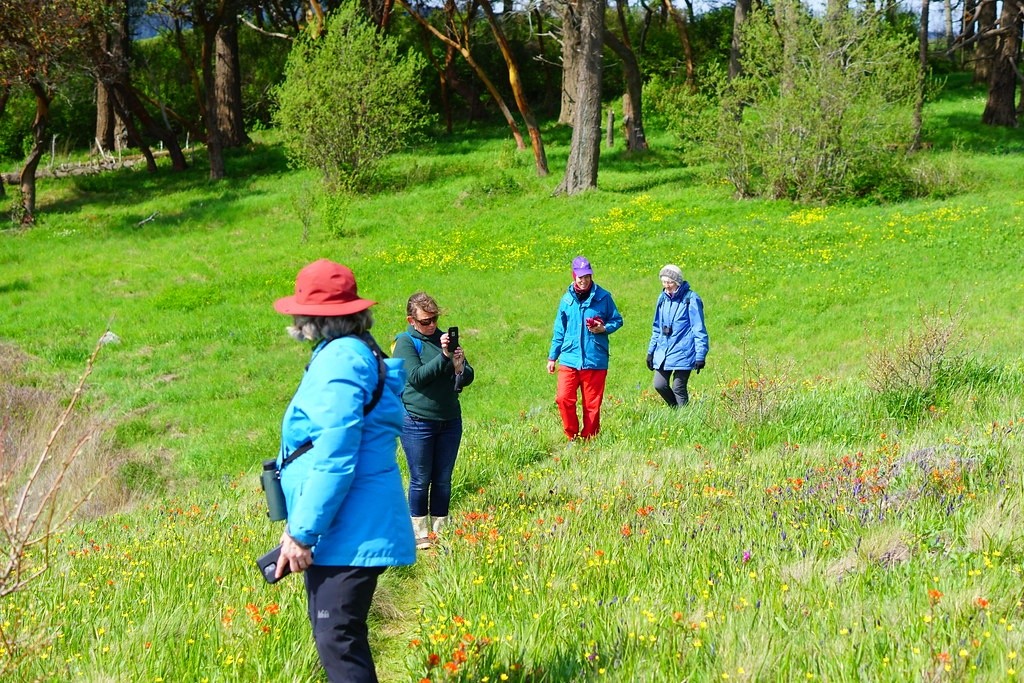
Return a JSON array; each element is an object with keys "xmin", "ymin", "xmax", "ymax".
[
  {"xmin": 448, "ymin": 327, "xmax": 458, "ymax": 352},
  {"xmin": 256, "ymin": 542, "xmax": 314, "ymax": 583}
]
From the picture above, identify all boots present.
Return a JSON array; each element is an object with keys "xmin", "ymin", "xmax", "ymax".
[
  {"xmin": 410, "ymin": 515, "xmax": 430, "ymax": 550},
  {"xmin": 429, "ymin": 516, "xmax": 449, "ymax": 535}
]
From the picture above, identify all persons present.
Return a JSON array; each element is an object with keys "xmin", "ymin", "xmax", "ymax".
[
  {"xmin": 546, "ymin": 256, "xmax": 624, "ymax": 450},
  {"xmin": 272, "ymin": 257, "xmax": 417, "ymax": 683},
  {"xmin": 646, "ymin": 264, "xmax": 709, "ymax": 412},
  {"xmin": 392, "ymin": 291, "xmax": 474, "ymax": 556}
]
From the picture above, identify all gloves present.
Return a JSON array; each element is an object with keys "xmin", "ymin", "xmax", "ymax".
[
  {"xmin": 646, "ymin": 354, "xmax": 655, "ymax": 371},
  {"xmin": 694, "ymin": 360, "xmax": 705, "ymax": 374}
]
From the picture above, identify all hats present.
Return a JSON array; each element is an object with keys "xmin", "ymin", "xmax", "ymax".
[
  {"xmin": 659, "ymin": 264, "xmax": 683, "ymax": 286},
  {"xmin": 572, "ymin": 257, "xmax": 593, "ymax": 277},
  {"xmin": 273, "ymin": 257, "xmax": 378, "ymax": 316}
]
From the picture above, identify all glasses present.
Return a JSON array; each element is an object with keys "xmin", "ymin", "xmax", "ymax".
[{"xmin": 412, "ymin": 315, "xmax": 439, "ymax": 326}]
